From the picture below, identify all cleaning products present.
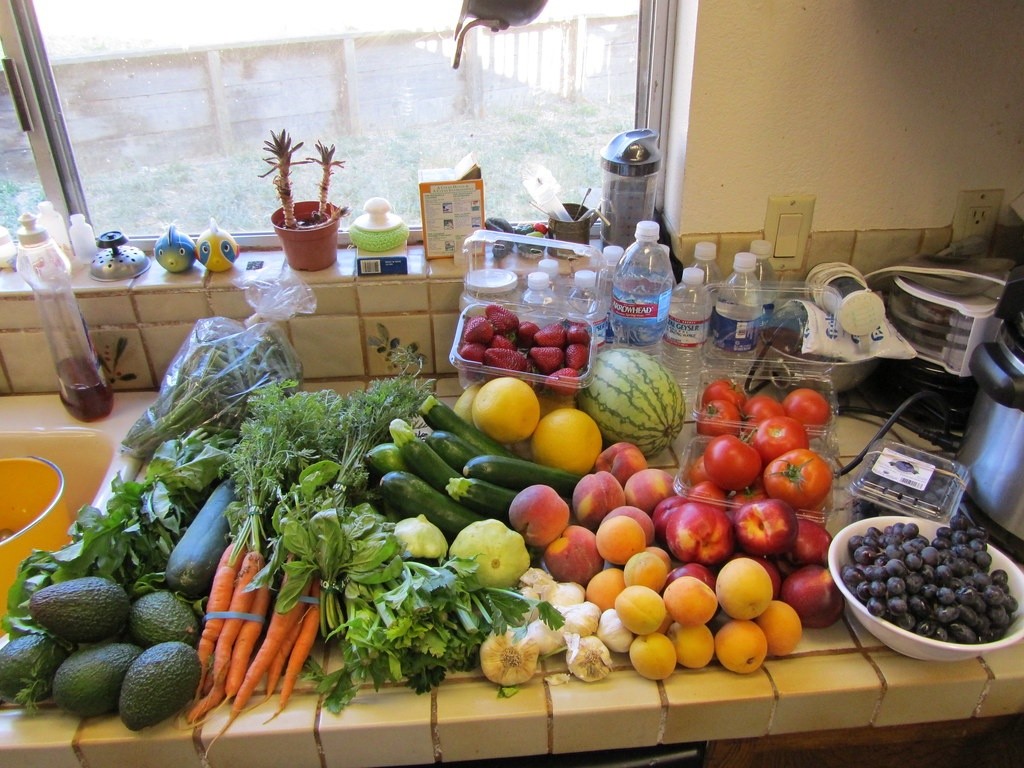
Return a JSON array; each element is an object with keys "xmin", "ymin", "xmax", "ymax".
[{"xmin": 15, "ymin": 211, "xmax": 115, "ymax": 423}]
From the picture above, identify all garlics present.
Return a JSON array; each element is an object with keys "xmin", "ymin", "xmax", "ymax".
[{"xmin": 481, "ymin": 569, "xmax": 633, "ymax": 686}]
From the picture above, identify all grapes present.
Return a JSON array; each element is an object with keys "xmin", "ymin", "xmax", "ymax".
[{"xmin": 840, "ymin": 513, "xmax": 1019, "ymax": 647}]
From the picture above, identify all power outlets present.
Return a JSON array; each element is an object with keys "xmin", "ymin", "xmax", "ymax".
[{"xmin": 953, "ymin": 189, "xmax": 1002, "ymax": 248}]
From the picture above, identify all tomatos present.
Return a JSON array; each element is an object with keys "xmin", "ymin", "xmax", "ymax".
[{"xmin": 681, "ymin": 376, "xmax": 834, "ymax": 526}]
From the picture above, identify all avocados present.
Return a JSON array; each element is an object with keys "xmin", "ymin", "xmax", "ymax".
[{"xmin": 0, "ymin": 575, "xmax": 202, "ymax": 732}]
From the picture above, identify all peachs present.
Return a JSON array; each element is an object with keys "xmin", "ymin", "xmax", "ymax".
[{"xmin": 508, "ymin": 442, "xmax": 847, "ymax": 630}]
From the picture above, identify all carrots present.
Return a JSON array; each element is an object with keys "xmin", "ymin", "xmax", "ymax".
[{"xmin": 186, "ymin": 542, "xmax": 320, "ymax": 757}]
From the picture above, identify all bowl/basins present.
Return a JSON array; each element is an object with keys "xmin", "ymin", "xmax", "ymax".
[
  {"xmin": 671, "ymin": 282, "xmax": 841, "ymax": 525},
  {"xmin": 0, "ymin": 456, "xmax": 73, "ymax": 636},
  {"xmin": 447, "ymin": 230, "xmax": 606, "ymax": 415},
  {"xmin": 826, "ymin": 516, "xmax": 1024, "ymax": 663},
  {"xmin": 845, "ymin": 439, "xmax": 970, "ymax": 524}
]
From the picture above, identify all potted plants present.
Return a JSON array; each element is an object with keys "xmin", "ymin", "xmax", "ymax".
[{"xmin": 260, "ymin": 130, "xmax": 343, "ymax": 272}]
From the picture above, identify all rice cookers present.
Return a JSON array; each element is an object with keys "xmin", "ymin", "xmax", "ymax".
[{"xmin": 954, "ymin": 304, "xmax": 1024, "ymax": 565}]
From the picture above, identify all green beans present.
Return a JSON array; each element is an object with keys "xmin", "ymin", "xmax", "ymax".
[{"xmin": 119, "ymin": 327, "xmax": 305, "ymax": 455}]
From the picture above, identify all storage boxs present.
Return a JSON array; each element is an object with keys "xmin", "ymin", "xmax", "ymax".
[
  {"xmin": 356, "ymin": 238, "xmax": 409, "ymax": 275},
  {"xmin": 451, "ymin": 230, "xmax": 609, "ymax": 403},
  {"xmin": 670, "ymin": 280, "xmax": 844, "ymax": 521},
  {"xmin": 848, "ymin": 438, "xmax": 969, "ymax": 524}
]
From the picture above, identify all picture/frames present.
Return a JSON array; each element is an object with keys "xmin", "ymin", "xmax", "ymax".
[{"xmin": 418, "ymin": 178, "xmax": 485, "ymax": 260}]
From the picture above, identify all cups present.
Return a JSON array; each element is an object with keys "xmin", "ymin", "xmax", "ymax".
[{"xmin": 547, "ymin": 203, "xmax": 591, "ymax": 258}]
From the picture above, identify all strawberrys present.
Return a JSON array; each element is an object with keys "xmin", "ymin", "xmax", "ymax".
[{"xmin": 457, "ymin": 305, "xmax": 591, "ymax": 396}]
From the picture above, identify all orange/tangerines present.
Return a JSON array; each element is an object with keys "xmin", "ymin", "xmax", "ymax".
[{"xmin": 453, "ymin": 374, "xmax": 604, "ymax": 476}]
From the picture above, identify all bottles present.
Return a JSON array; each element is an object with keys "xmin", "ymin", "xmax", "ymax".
[
  {"xmin": 36, "ymin": 202, "xmax": 71, "ymax": 259},
  {"xmin": 458, "ymin": 220, "xmax": 676, "ymax": 371},
  {"xmin": 659, "ymin": 267, "xmax": 712, "ymax": 404},
  {"xmin": 707, "ymin": 251, "xmax": 764, "ymax": 371},
  {"xmin": 69, "ymin": 213, "xmax": 97, "ymax": 257},
  {"xmin": 747, "ymin": 238, "xmax": 780, "ymax": 307},
  {"xmin": 0, "ymin": 225, "xmax": 17, "ymax": 269},
  {"xmin": 599, "ymin": 127, "xmax": 662, "ymax": 250},
  {"xmin": 15, "ymin": 211, "xmax": 114, "ymax": 423},
  {"xmin": 689, "ymin": 240, "xmax": 724, "ymax": 293}
]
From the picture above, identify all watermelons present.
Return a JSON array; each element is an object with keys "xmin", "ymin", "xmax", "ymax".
[{"xmin": 576, "ymin": 348, "xmax": 686, "ymax": 453}]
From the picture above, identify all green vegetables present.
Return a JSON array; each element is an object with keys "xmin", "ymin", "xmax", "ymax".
[
  {"xmin": 1, "ymin": 426, "xmax": 232, "ymax": 716},
  {"xmin": 239, "ymin": 459, "xmax": 567, "ymax": 715}
]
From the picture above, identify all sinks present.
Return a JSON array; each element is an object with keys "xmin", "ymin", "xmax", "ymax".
[{"xmin": 0, "ymin": 423, "xmax": 144, "ymax": 653}]
